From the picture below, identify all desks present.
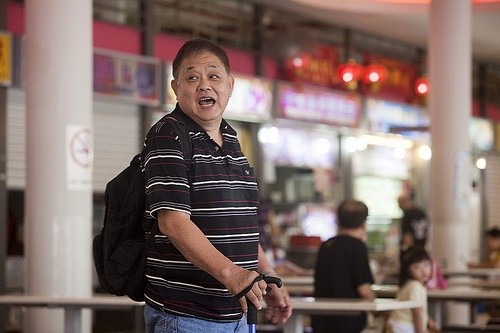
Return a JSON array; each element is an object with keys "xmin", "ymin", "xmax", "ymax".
[
  {"xmin": 281, "ymin": 268, "xmax": 500, "ymax": 333},
  {"xmin": 0, "ymin": 296, "xmax": 145, "ymax": 333}
]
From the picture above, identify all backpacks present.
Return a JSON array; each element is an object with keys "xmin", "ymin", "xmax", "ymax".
[{"xmin": 93, "ymin": 114, "xmax": 193, "ymax": 302}]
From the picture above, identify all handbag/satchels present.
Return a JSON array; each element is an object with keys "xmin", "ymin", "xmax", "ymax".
[{"xmin": 427, "ymin": 257, "xmax": 446, "ymax": 289}]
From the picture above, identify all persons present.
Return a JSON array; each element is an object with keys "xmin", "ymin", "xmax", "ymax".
[
  {"xmin": 140, "ymin": 40, "xmax": 292, "ymax": 333},
  {"xmin": 265, "ymin": 190, "xmax": 500, "ymax": 333}
]
